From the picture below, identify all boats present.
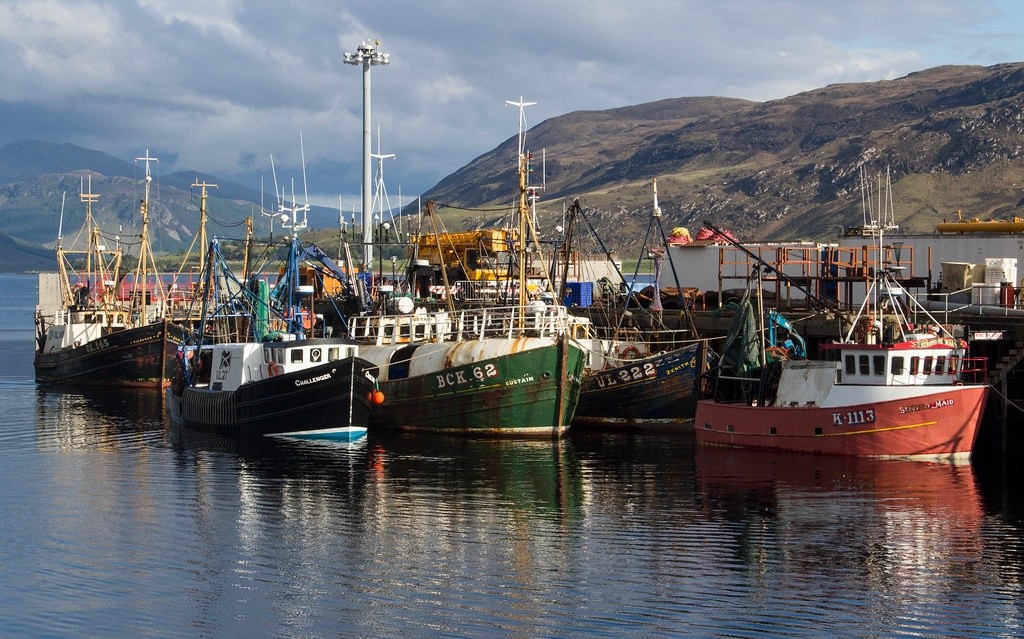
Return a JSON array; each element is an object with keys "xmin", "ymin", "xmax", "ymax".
[
  {"xmin": 696, "ymin": 445, "xmax": 980, "ymax": 545},
  {"xmin": 166, "ymin": 130, "xmax": 380, "ymax": 454},
  {"xmin": 694, "ymin": 162, "xmax": 991, "ymax": 465}
]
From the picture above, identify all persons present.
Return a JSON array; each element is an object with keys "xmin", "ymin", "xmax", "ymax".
[
  {"xmin": 76, "ymin": 283, "xmax": 90, "ymax": 310},
  {"xmin": 913, "ymin": 324, "xmax": 919, "ymax": 334}
]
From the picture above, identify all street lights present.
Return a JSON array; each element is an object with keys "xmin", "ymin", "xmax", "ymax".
[{"xmin": 343, "ymin": 40, "xmax": 390, "ymax": 274}]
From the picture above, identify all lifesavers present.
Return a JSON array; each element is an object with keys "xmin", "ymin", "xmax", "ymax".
[{"xmin": 621, "ymin": 346, "xmax": 640, "ymax": 364}]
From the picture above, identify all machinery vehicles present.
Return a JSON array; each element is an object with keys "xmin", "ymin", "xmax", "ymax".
[{"xmin": 407, "ymin": 228, "xmax": 525, "ymax": 298}]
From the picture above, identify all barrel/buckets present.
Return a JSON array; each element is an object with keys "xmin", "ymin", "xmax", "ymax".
[{"xmin": 1000, "ymin": 281, "xmax": 1015, "ymax": 308}]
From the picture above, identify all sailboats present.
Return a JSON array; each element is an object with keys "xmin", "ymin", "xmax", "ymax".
[{"xmin": 35, "ymin": 95, "xmax": 709, "ymax": 437}]
